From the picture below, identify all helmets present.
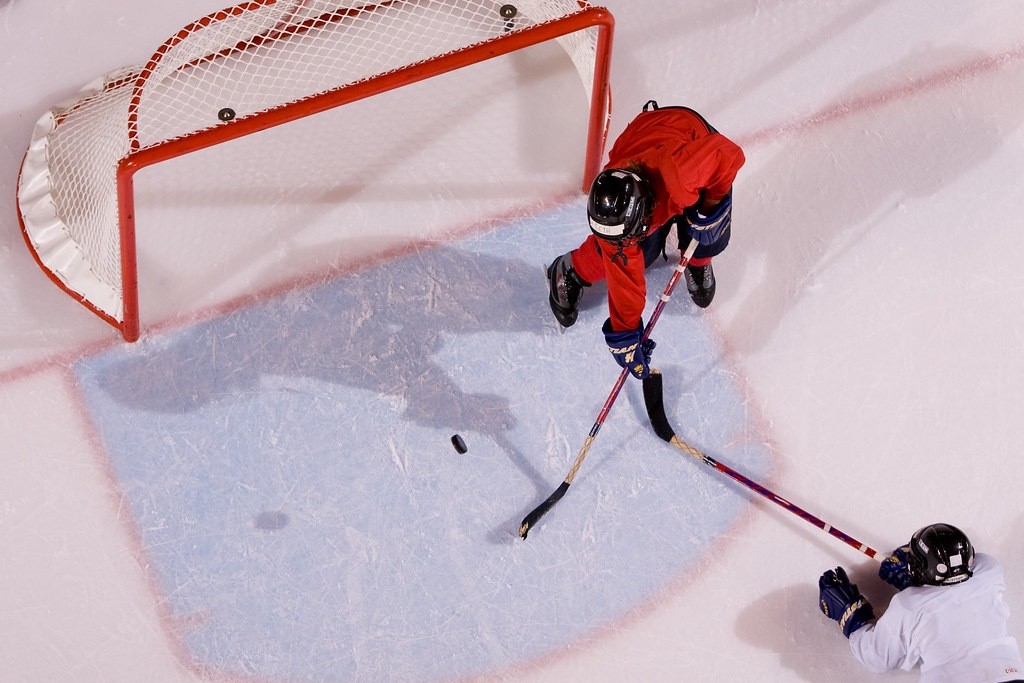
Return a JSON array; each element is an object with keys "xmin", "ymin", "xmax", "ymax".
[
  {"xmin": 587, "ymin": 166, "xmax": 649, "ymax": 241},
  {"xmin": 908, "ymin": 522, "xmax": 975, "ymax": 587}
]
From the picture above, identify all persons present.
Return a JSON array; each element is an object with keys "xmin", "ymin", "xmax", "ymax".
[
  {"xmin": 543, "ymin": 105, "xmax": 746, "ymax": 380},
  {"xmin": 819, "ymin": 523, "xmax": 1024, "ymax": 683}
]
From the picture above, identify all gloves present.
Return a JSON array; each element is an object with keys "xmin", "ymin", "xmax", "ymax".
[
  {"xmin": 880, "ymin": 544, "xmax": 916, "ymax": 588},
  {"xmin": 601, "ymin": 313, "xmax": 657, "ymax": 379},
  {"xmin": 685, "ymin": 195, "xmax": 733, "ymax": 248},
  {"xmin": 818, "ymin": 565, "xmax": 877, "ymax": 638}
]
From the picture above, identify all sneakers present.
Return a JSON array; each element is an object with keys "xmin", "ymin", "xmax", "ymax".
[
  {"xmin": 542, "ymin": 249, "xmax": 592, "ymax": 334},
  {"xmin": 680, "ymin": 249, "xmax": 716, "ymax": 310}
]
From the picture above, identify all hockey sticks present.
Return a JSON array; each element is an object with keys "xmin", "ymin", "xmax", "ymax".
[
  {"xmin": 517, "ymin": 237, "xmax": 701, "ymax": 537},
  {"xmin": 642, "ymin": 367, "xmax": 887, "ymax": 563}
]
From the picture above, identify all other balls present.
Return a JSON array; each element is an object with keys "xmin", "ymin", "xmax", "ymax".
[{"xmin": 451, "ymin": 433, "xmax": 467, "ymax": 456}]
